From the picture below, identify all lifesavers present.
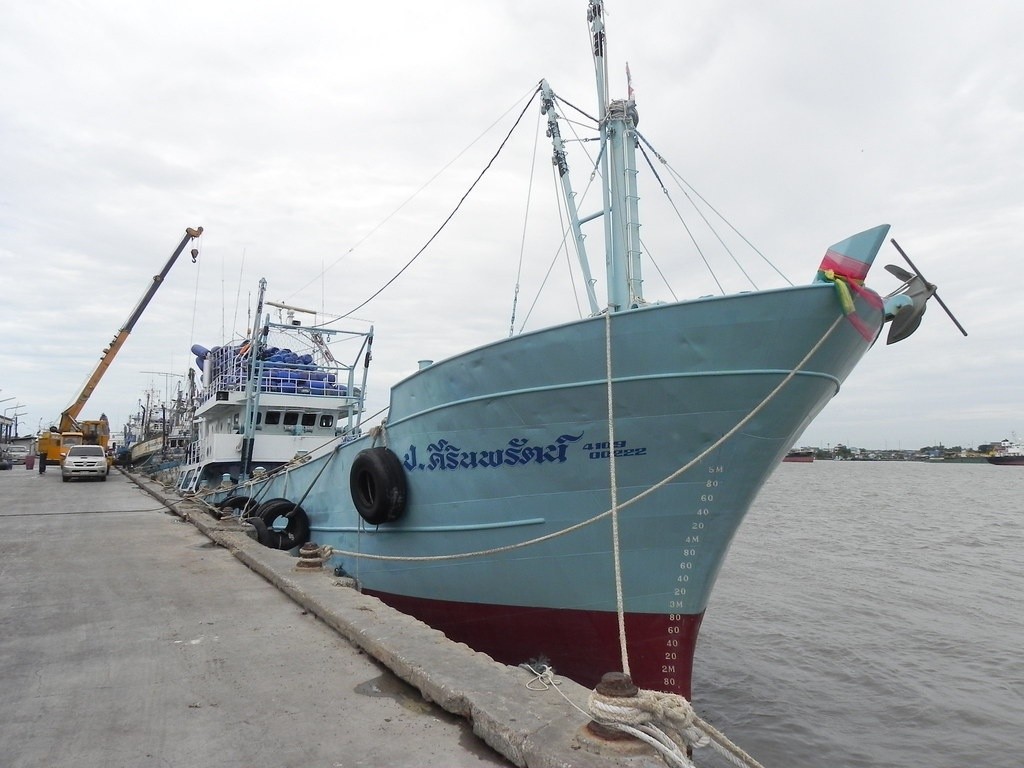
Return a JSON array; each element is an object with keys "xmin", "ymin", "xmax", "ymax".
[
  {"xmin": 214, "ymin": 496, "xmax": 308, "ymax": 551},
  {"xmin": 350, "ymin": 447, "xmax": 408, "ymax": 526}
]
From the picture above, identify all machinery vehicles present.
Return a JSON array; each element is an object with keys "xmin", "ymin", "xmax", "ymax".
[{"xmin": 35, "ymin": 226, "xmax": 205, "ymax": 474}]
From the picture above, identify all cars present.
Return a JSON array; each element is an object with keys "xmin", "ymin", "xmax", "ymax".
[
  {"xmin": 3, "ymin": 445, "xmax": 29, "ymax": 465},
  {"xmin": 59, "ymin": 445, "xmax": 109, "ymax": 483}
]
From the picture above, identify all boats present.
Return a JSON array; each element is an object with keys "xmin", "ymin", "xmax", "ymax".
[
  {"xmin": 120, "ymin": 0, "xmax": 967, "ymax": 707},
  {"xmin": 783, "ymin": 450, "xmax": 814, "ymax": 463},
  {"xmin": 986, "ymin": 450, "xmax": 1024, "ymax": 466}
]
